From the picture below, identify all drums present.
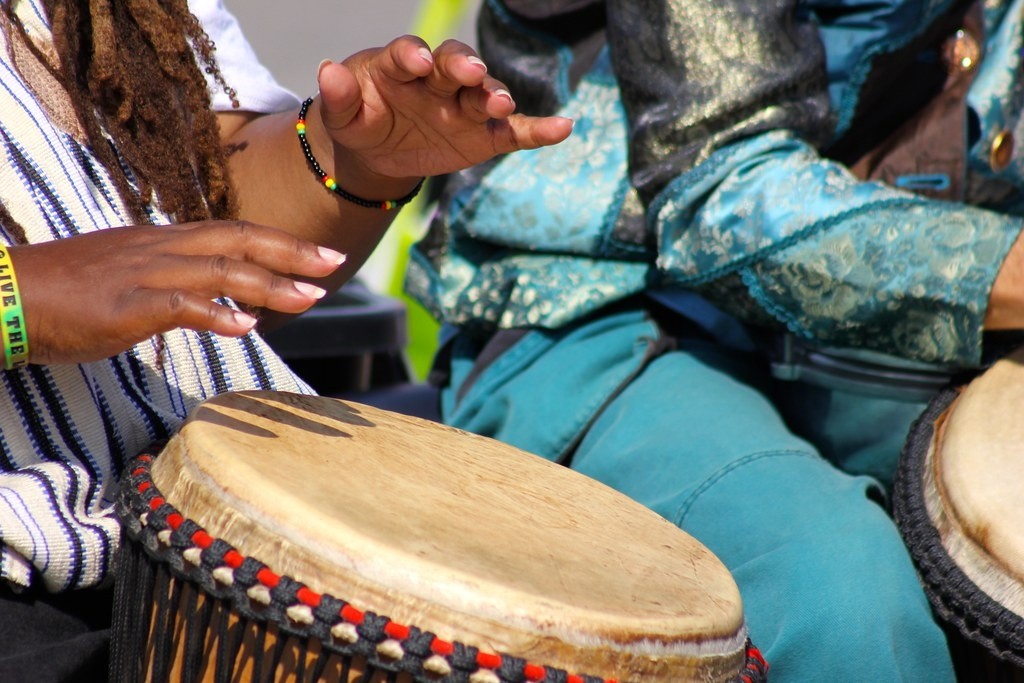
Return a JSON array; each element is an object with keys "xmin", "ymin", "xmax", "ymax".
[
  {"xmin": 105, "ymin": 387, "xmax": 766, "ymax": 683},
  {"xmin": 894, "ymin": 344, "xmax": 1024, "ymax": 683}
]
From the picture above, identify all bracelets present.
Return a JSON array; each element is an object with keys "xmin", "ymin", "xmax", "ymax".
[
  {"xmin": 298, "ymin": 96, "xmax": 424, "ymax": 210},
  {"xmin": 0, "ymin": 246, "xmax": 27, "ymax": 371}
]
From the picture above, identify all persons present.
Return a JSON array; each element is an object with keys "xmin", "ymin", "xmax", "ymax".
[
  {"xmin": 405, "ymin": 0, "xmax": 1024, "ymax": 683},
  {"xmin": 0, "ymin": 0, "xmax": 572, "ymax": 683}
]
